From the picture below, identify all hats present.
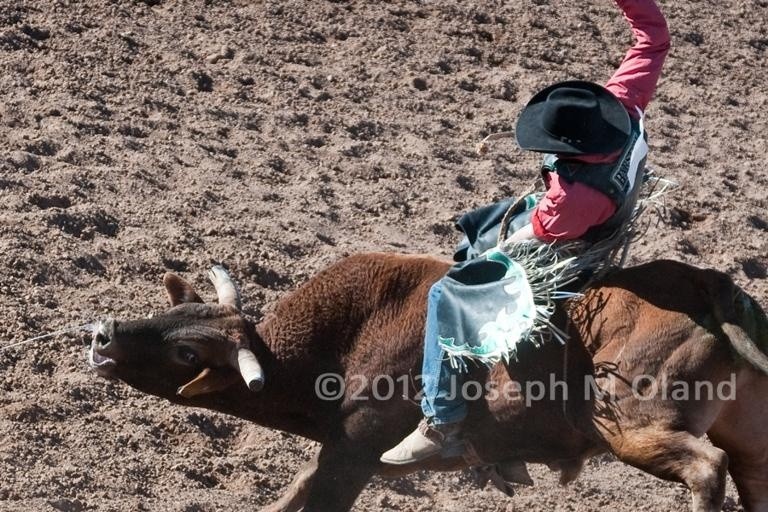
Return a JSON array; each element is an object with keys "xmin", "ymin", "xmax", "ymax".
[{"xmin": 516, "ymin": 81, "xmax": 631, "ymax": 157}]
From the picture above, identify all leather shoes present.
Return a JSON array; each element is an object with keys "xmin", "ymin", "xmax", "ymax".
[{"xmin": 380, "ymin": 416, "xmax": 471, "ymax": 465}]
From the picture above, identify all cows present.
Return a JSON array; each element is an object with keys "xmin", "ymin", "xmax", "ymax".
[{"xmin": 87, "ymin": 252, "xmax": 767, "ymax": 512}]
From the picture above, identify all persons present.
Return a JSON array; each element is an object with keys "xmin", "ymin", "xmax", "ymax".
[{"xmin": 377, "ymin": 1, "xmax": 675, "ymax": 471}]
